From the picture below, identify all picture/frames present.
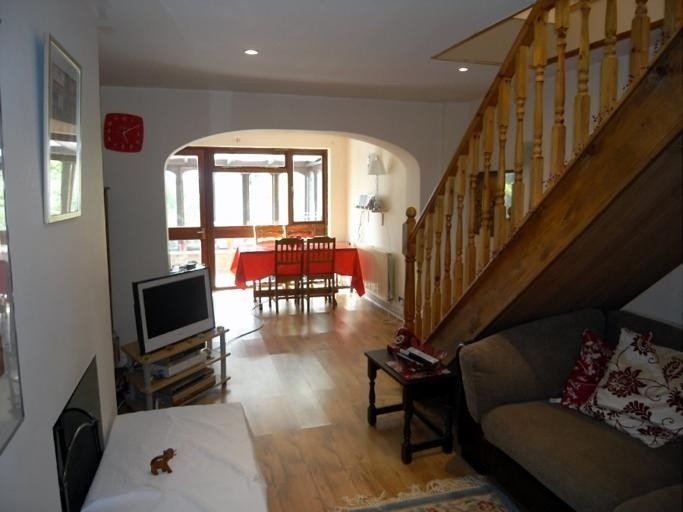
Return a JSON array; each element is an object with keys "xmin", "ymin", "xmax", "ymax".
[{"xmin": 37, "ymin": 29, "xmax": 92, "ymax": 224}]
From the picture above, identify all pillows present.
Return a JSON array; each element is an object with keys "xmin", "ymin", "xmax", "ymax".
[
  {"xmin": 576, "ymin": 326, "xmax": 682, "ymax": 451},
  {"xmin": 548, "ymin": 326, "xmax": 654, "ymax": 413}
]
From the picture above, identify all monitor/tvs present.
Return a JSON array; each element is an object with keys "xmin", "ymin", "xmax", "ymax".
[{"xmin": 132, "ymin": 265, "xmax": 215, "ymax": 355}]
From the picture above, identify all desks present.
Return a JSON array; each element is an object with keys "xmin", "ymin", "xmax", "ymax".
[{"xmin": 361, "ymin": 345, "xmax": 455, "ymax": 464}]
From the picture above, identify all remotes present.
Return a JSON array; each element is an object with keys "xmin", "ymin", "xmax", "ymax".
[{"xmin": 186, "ymin": 261, "xmax": 197, "ymax": 269}]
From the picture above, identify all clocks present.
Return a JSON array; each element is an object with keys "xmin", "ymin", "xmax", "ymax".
[{"xmin": 103, "ymin": 110, "xmax": 146, "ymax": 155}]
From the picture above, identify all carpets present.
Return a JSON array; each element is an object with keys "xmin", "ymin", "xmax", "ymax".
[{"xmin": 333, "ymin": 473, "xmax": 520, "ymax": 512}]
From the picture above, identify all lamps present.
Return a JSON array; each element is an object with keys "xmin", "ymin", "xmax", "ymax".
[{"xmin": 363, "ymin": 149, "xmax": 386, "ymax": 208}]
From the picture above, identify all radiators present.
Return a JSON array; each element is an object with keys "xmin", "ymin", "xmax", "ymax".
[{"xmin": 354, "ymin": 244, "xmax": 395, "ymax": 302}]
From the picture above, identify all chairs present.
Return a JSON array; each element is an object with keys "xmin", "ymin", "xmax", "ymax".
[{"xmin": 227, "ymin": 224, "xmax": 365, "ymax": 315}]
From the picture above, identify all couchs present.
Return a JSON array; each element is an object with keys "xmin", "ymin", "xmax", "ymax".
[{"xmin": 449, "ymin": 303, "xmax": 683, "ymax": 512}]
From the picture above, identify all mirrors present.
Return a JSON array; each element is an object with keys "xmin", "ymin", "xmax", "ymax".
[{"xmin": 0, "ymin": 94, "xmax": 28, "ymax": 460}]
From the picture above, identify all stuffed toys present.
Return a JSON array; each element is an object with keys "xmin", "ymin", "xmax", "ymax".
[{"xmin": 150, "ymin": 448, "xmax": 177, "ymax": 474}]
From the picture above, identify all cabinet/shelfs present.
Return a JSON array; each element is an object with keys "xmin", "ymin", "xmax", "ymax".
[{"xmin": 110, "ymin": 325, "xmax": 235, "ymax": 414}]
